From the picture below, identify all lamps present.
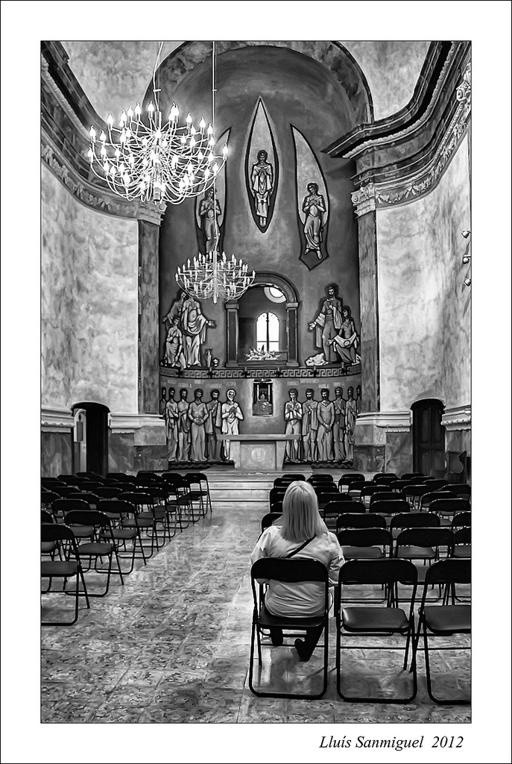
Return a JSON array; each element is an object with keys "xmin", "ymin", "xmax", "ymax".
[
  {"xmin": 85, "ymin": 41, "xmax": 231, "ymax": 213},
  {"xmin": 173, "ymin": 214, "xmax": 256, "ymax": 303}
]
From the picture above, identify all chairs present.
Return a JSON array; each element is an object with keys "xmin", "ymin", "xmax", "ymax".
[{"xmin": 41, "ymin": 471, "xmax": 213, "ymax": 627}]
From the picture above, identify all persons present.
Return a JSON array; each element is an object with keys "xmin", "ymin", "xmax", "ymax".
[
  {"xmin": 199, "ymin": 186, "xmax": 222, "ymax": 256},
  {"xmin": 250, "ymin": 149, "xmax": 274, "ymax": 227},
  {"xmin": 307, "ymin": 284, "xmax": 343, "ymax": 364},
  {"xmin": 182, "ymin": 295, "xmax": 217, "ymax": 368},
  {"xmin": 251, "ymin": 479, "xmax": 344, "ymax": 661},
  {"xmin": 161, "ymin": 289, "xmax": 189, "ymax": 329},
  {"xmin": 327, "ymin": 304, "xmax": 361, "ymax": 365},
  {"xmin": 302, "ymin": 182, "xmax": 326, "ymax": 260},
  {"xmin": 161, "ymin": 315, "xmax": 186, "ymax": 370},
  {"xmin": 159, "ymin": 381, "xmax": 361, "ymax": 464}
]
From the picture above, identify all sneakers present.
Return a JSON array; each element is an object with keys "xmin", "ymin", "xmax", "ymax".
[
  {"xmin": 272, "ymin": 637, "xmax": 284, "ymax": 648},
  {"xmin": 295, "ymin": 639, "xmax": 308, "ymax": 662}
]
